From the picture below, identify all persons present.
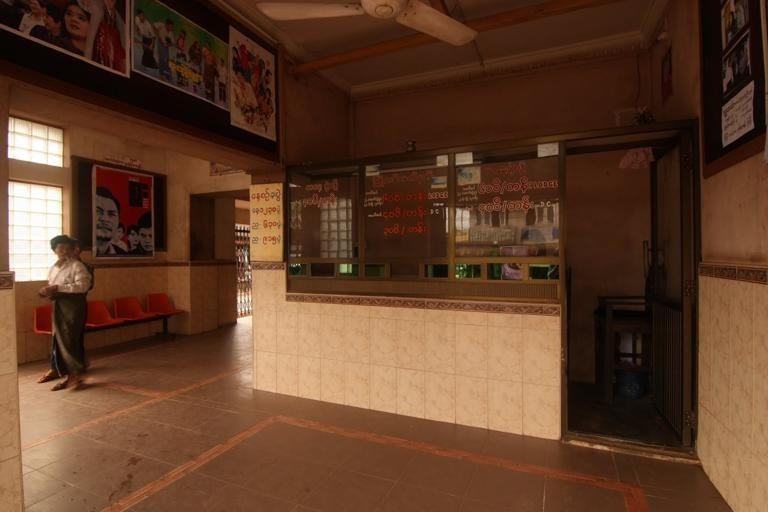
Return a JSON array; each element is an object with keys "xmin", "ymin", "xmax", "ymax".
[
  {"xmin": 125, "ymin": 224, "xmax": 140, "ymax": 251},
  {"xmin": 110, "ymin": 222, "xmax": 128, "ymax": 252},
  {"xmin": 1, "ymin": 0, "xmax": 276, "ymax": 129},
  {"xmin": 124, "ymin": 212, "xmax": 152, "ymax": 256},
  {"xmin": 94, "ymin": 183, "xmax": 127, "ymax": 255},
  {"xmin": 721, "ymin": 1, "xmax": 752, "ymax": 92}
]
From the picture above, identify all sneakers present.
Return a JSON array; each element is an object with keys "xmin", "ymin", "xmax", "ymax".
[{"xmin": 35, "ymin": 374, "xmax": 70, "ymax": 392}]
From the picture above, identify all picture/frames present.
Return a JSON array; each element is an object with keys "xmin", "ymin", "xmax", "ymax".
[
  {"xmin": 2, "ymin": 2, "xmax": 132, "ymax": 79},
  {"xmin": 229, "ymin": 24, "xmax": 278, "ymax": 141},
  {"xmin": 131, "ymin": 2, "xmax": 231, "ymax": 110}
]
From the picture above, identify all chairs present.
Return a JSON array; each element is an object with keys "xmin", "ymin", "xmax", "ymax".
[
  {"xmin": 594, "ymin": 270, "xmax": 651, "ymax": 405},
  {"xmin": 33, "ymin": 293, "xmax": 185, "ymax": 335}
]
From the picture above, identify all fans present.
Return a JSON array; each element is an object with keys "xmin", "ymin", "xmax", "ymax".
[{"xmin": 258, "ymin": 0, "xmax": 477, "ymax": 49}]
